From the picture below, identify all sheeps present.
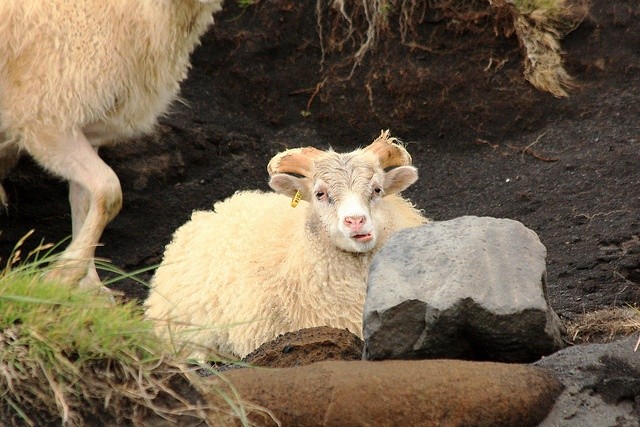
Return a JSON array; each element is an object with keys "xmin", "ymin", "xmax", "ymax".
[
  {"xmin": 1, "ymin": 0, "xmax": 222, "ymax": 306},
  {"xmin": 142, "ymin": 129, "xmax": 434, "ymax": 364}
]
